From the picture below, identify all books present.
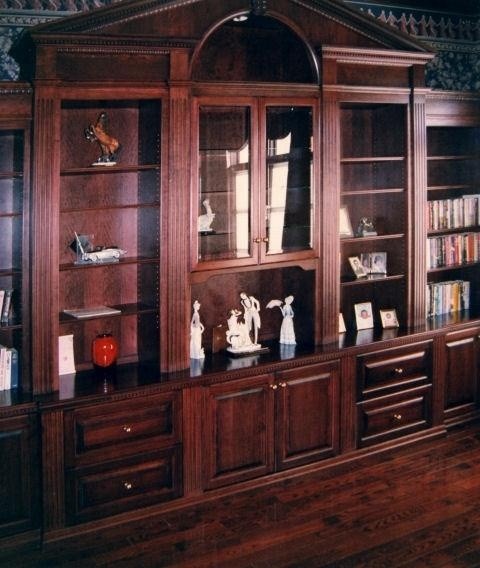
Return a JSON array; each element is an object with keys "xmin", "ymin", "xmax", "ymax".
[
  {"xmin": 0, "ymin": 289, "xmax": 13, "ymax": 323},
  {"xmin": 64, "ymin": 305, "xmax": 121, "ymax": 319},
  {"xmin": 426, "ymin": 194, "xmax": 480, "ymax": 269},
  {"xmin": 0, "ymin": 344, "xmax": 18, "ymax": 391},
  {"xmin": 426, "ymin": 280, "xmax": 470, "ymax": 317}
]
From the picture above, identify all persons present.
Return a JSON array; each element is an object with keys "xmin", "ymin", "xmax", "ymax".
[
  {"xmin": 227, "ymin": 291, "xmax": 261, "ymax": 347},
  {"xmin": 373, "ymin": 256, "xmax": 386, "ymax": 272},
  {"xmin": 358, "ymin": 310, "xmax": 373, "ymax": 328},
  {"xmin": 353, "ymin": 260, "xmax": 363, "ymax": 273},
  {"xmin": 279, "ymin": 295, "xmax": 297, "ymax": 344},
  {"xmin": 190, "ymin": 300, "xmax": 205, "ymax": 358}
]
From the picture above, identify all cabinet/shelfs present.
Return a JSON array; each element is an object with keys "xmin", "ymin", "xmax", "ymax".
[
  {"xmin": 0, "ymin": 82, "xmax": 31, "ymax": 392},
  {"xmin": 1, "ymin": 401, "xmax": 42, "ymax": 556},
  {"xmin": 322, "ymin": 83, "xmax": 426, "ymax": 344},
  {"xmin": 29, "ymin": 80, "xmax": 191, "ymax": 395},
  {"xmin": 189, "ymin": 351, "xmax": 341, "ymax": 507},
  {"xmin": 337, "ymin": 333, "xmax": 449, "ymax": 466},
  {"xmin": 38, "ymin": 379, "xmax": 193, "ymax": 547},
  {"xmin": 412, "ymin": 87, "xmax": 480, "ymax": 330},
  {"xmin": 186, "ymin": 82, "xmax": 339, "ymax": 368},
  {"xmin": 439, "ymin": 323, "xmax": 480, "ymax": 433}
]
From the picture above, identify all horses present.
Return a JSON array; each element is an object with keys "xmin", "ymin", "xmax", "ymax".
[{"xmin": 83, "ymin": 112, "xmax": 122, "ymax": 162}]
